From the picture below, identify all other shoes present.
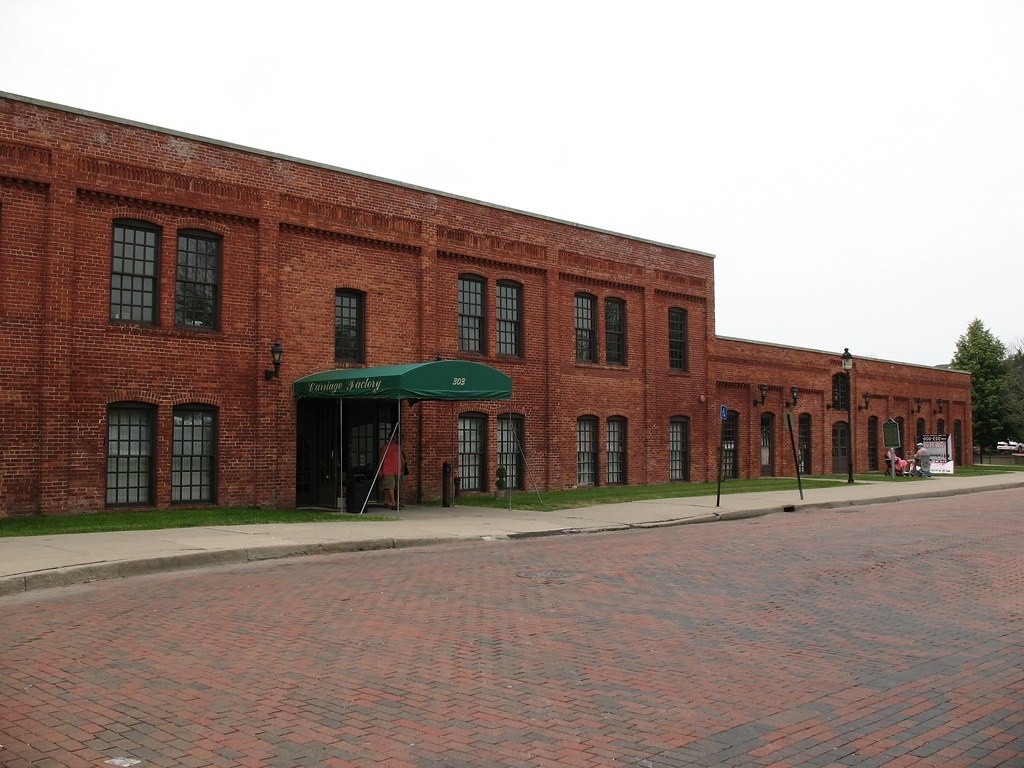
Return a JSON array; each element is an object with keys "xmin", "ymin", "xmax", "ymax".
[{"xmin": 384, "ymin": 503, "xmax": 400, "ymax": 510}]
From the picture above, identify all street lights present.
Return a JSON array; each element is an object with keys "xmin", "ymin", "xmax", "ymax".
[{"xmin": 841, "ymin": 347, "xmax": 856, "ymax": 484}]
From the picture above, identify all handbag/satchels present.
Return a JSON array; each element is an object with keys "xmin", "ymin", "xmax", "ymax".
[{"xmin": 403, "ymin": 462, "xmax": 410, "ymax": 476}]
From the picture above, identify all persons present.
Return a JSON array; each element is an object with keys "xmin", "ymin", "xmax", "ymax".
[
  {"xmin": 886, "ymin": 443, "xmax": 927, "ymax": 477},
  {"xmin": 378, "ymin": 432, "xmax": 408, "ymax": 510}
]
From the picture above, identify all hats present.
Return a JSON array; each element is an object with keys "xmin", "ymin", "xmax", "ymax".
[{"xmin": 916, "ymin": 443, "xmax": 923, "ymax": 446}]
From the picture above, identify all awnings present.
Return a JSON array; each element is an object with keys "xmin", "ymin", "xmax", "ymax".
[{"xmin": 292, "ymin": 360, "xmax": 511, "ymax": 517}]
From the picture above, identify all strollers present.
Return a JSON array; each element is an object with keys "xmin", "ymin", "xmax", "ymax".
[{"xmin": 884, "ymin": 453, "xmax": 902, "ymax": 477}]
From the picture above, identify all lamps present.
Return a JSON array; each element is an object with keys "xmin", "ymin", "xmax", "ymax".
[
  {"xmin": 266, "ymin": 340, "xmax": 284, "ymax": 379},
  {"xmin": 753, "ymin": 383, "xmax": 769, "ymax": 406},
  {"xmin": 910, "ymin": 398, "xmax": 922, "ymax": 415},
  {"xmin": 786, "ymin": 386, "xmax": 800, "ymax": 408},
  {"xmin": 934, "ymin": 399, "xmax": 945, "ymax": 415},
  {"xmin": 858, "ymin": 392, "xmax": 872, "ymax": 411}
]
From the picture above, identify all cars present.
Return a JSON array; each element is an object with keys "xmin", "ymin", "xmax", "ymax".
[{"xmin": 998, "ymin": 439, "xmax": 1024, "ymax": 452}]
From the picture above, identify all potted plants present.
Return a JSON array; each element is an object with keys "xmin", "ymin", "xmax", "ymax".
[{"xmin": 495, "ymin": 466, "xmax": 508, "ymax": 498}]
